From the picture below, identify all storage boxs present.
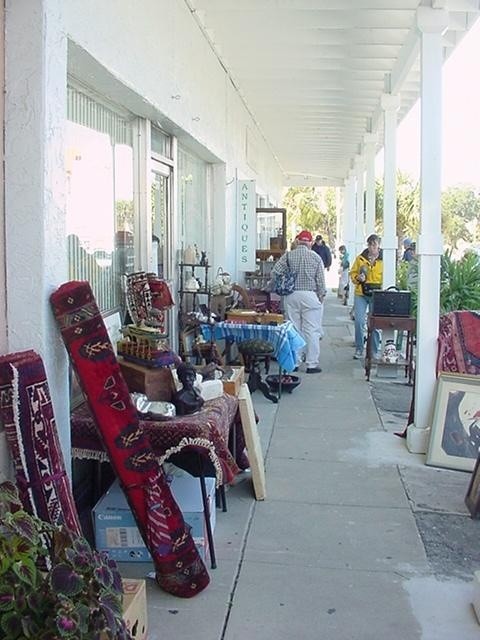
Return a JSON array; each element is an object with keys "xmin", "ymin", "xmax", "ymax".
[
  {"xmin": 43, "ymin": 573, "xmax": 148, "ymax": 640},
  {"xmin": 91, "ymin": 463, "xmax": 218, "ymax": 564}
]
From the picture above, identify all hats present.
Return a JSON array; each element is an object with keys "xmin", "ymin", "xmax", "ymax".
[
  {"xmin": 314, "ymin": 235, "xmax": 323, "ymax": 241},
  {"xmin": 297, "ymin": 230, "xmax": 313, "ymax": 242},
  {"xmin": 404, "ymin": 239, "xmax": 412, "ymax": 244}
]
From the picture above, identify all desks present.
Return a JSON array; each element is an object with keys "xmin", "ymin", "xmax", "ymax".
[
  {"xmin": 69, "ymin": 390, "xmax": 236, "ymax": 570},
  {"xmin": 365, "ymin": 315, "xmax": 418, "ymax": 386},
  {"xmin": 217, "ymin": 318, "xmax": 306, "ymax": 399}
]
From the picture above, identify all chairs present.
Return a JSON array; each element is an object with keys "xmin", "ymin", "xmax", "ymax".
[{"xmin": 249, "ymin": 289, "xmax": 273, "ymax": 310}]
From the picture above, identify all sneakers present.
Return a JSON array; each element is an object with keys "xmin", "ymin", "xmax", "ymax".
[
  {"xmin": 354, "ymin": 350, "xmax": 365, "ymax": 360},
  {"xmin": 293, "ymin": 367, "xmax": 300, "ymax": 372},
  {"xmin": 306, "ymin": 366, "xmax": 321, "ymax": 373}
]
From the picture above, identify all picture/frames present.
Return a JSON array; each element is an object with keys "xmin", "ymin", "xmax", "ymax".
[
  {"xmin": 424, "ymin": 370, "xmax": 480, "ymax": 472},
  {"xmin": 463, "ymin": 452, "xmax": 480, "ymax": 519}
]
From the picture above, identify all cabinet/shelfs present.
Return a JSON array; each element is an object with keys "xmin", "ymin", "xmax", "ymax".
[
  {"xmin": 240, "ymin": 237, "xmax": 295, "ymax": 287},
  {"xmin": 179, "ymin": 263, "xmax": 211, "ymax": 335}
]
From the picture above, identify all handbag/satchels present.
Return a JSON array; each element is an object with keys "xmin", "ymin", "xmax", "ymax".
[
  {"xmin": 274, "ymin": 251, "xmax": 297, "ymax": 296},
  {"xmin": 370, "ymin": 286, "xmax": 412, "ymax": 318},
  {"xmin": 362, "ymin": 282, "xmax": 382, "ymax": 296}
]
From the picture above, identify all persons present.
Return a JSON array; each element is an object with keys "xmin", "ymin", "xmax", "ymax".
[
  {"xmin": 271, "ymin": 230, "xmax": 327, "ymax": 373},
  {"xmin": 173, "ymin": 361, "xmax": 205, "ymax": 415},
  {"xmin": 350, "ymin": 235, "xmax": 384, "ymax": 359},
  {"xmin": 403, "ymin": 237, "xmax": 413, "ymax": 262},
  {"xmin": 311, "ymin": 235, "xmax": 332, "ymax": 271},
  {"xmin": 339, "ymin": 245, "xmax": 350, "ymax": 299}
]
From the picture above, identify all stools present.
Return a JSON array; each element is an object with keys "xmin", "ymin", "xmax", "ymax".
[{"xmin": 238, "ymin": 340, "xmax": 278, "ymax": 404}]
